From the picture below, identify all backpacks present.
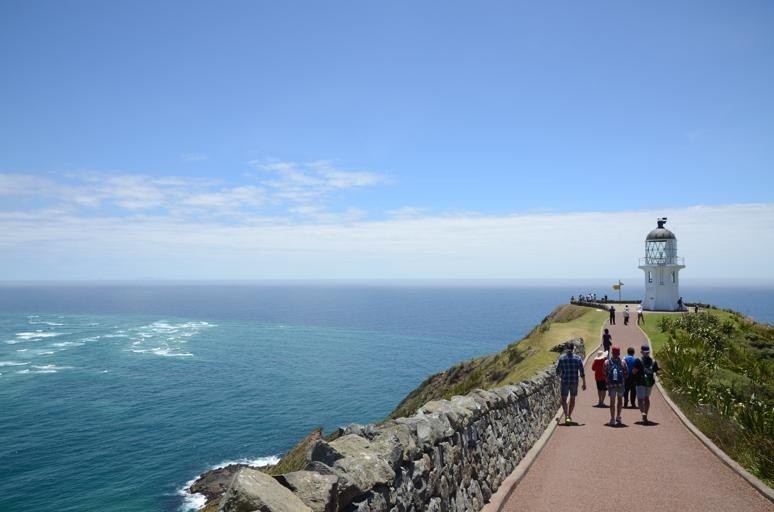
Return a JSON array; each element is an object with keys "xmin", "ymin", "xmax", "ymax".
[
  {"xmin": 639, "ymin": 356, "xmax": 655, "ymax": 386},
  {"xmin": 609, "ymin": 359, "xmax": 623, "ymax": 384}
]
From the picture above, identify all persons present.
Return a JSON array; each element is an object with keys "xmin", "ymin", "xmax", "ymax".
[
  {"xmin": 631, "ymin": 343, "xmax": 659, "ymax": 424},
  {"xmin": 570, "ymin": 292, "xmax": 607, "ymax": 303},
  {"xmin": 556, "ymin": 343, "xmax": 586, "ymax": 424},
  {"xmin": 623, "ymin": 308, "xmax": 629, "ymax": 325},
  {"xmin": 608, "ymin": 305, "xmax": 616, "ymax": 325},
  {"xmin": 635, "ymin": 305, "xmax": 645, "ymax": 325},
  {"xmin": 602, "ymin": 345, "xmax": 628, "ymax": 426},
  {"xmin": 603, "ymin": 328, "xmax": 612, "ymax": 352},
  {"xmin": 625, "ymin": 305, "xmax": 629, "ymax": 311},
  {"xmin": 622, "ymin": 347, "xmax": 639, "ymax": 408},
  {"xmin": 677, "ymin": 297, "xmax": 682, "ymax": 311},
  {"xmin": 591, "ymin": 349, "xmax": 609, "ymax": 406}
]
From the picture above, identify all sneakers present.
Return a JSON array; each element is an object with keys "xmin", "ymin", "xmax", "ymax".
[
  {"xmin": 608, "ymin": 417, "xmax": 621, "ymax": 426},
  {"xmin": 642, "ymin": 412, "xmax": 648, "ymax": 425},
  {"xmin": 565, "ymin": 417, "xmax": 572, "ymax": 424}
]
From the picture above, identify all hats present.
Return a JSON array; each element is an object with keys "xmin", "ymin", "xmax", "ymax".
[
  {"xmin": 641, "ymin": 345, "xmax": 650, "ymax": 353},
  {"xmin": 612, "ymin": 345, "xmax": 620, "ymax": 356},
  {"xmin": 594, "ymin": 350, "xmax": 608, "ymax": 360}
]
